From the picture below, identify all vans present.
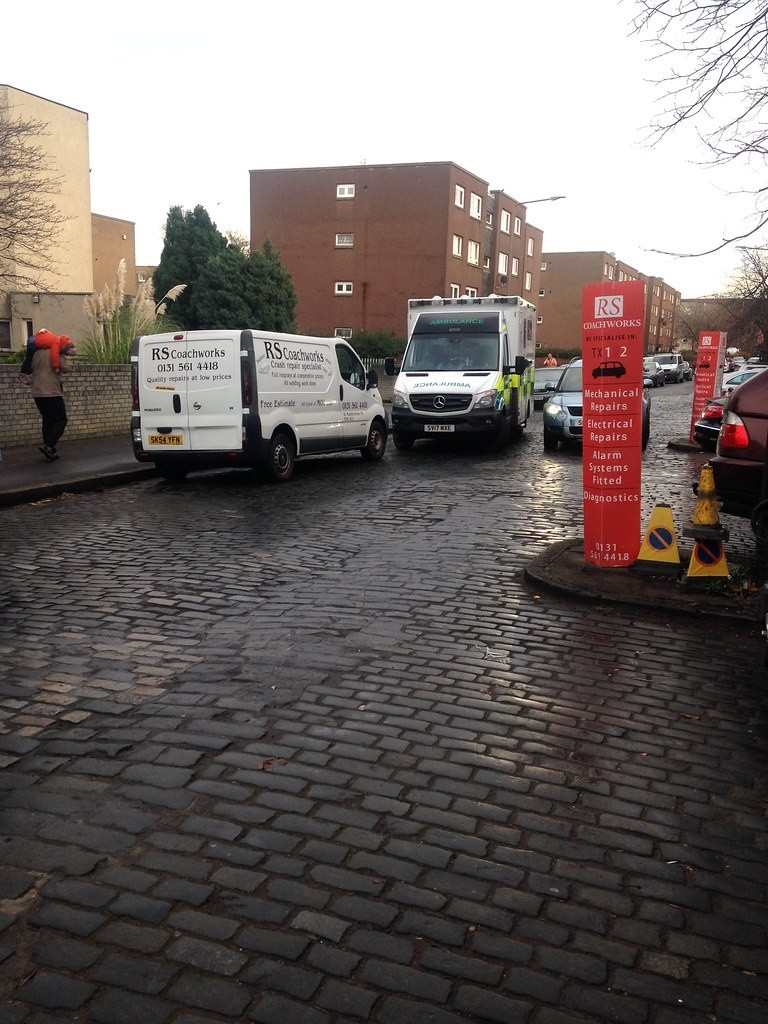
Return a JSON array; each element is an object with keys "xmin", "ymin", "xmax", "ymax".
[{"xmin": 130, "ymin": 329, "xmax": 389, "ymax": 480}]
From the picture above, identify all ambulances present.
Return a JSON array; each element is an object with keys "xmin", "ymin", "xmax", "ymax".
[{"xmin": 391, "ymin": 296, "xmax": 537, "ymax": 451}]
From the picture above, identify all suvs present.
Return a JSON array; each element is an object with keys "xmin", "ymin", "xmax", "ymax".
[{"xmin": 542, "ymin": 356, "xmax": 654, "ymax": 451}]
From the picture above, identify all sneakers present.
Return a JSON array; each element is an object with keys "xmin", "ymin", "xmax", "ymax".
[{"xmin": 38, "ymin": 444, "xmax": 58, "ymax": 461}]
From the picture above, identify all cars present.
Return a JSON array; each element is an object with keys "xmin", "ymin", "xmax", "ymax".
[
  {"xmin": 722, "ymin": 370, "xmax": 761, "ymax": 397},
  {"xmin": 683, "ymin": 361, "xmax": 693, "ymax": 381},
  {"xmin": 723, "ymin": 358, "xmax": 735, "ymax": 372},
  {"xmin": 693, "ymin": 397, "xmax": 730, "ymax": 454},
  {"xmin": 692, "ymin": 368, "xmax": 767, "ymax": 544},
  {"xmin": 534, "ymin": 367, "xmax": 565, "ymax": 405},
  {"xmin": 733, "ymin": 357, "xmax": 746, "ymax": 367},
  {"xmin": 653, "ymin": 354, "xmax": 684, "ymax": 383},
  {"xmin": 747, "ymin": 357, "xmax": 768, "ymax": 365},
  {"xmin": 643, "ymin": 362, "xmax": 665, "ymax": 387}
]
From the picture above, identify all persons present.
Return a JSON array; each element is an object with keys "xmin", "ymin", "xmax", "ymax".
[
  {"xmin": 16, "ymin": 332, "xmax": 77, "ymax": 384},
  {"xmin": 31, "ymin": 328, "xmax": 70, "ymax": 460},
  {"xmin": 544, "ymin": 354, "xmax": 557, "ymax": 367}
]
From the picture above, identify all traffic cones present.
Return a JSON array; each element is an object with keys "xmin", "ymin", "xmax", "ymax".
[{"xmin": 682, "ymin": 466, "xmax": 730, "ymax": 543}]
